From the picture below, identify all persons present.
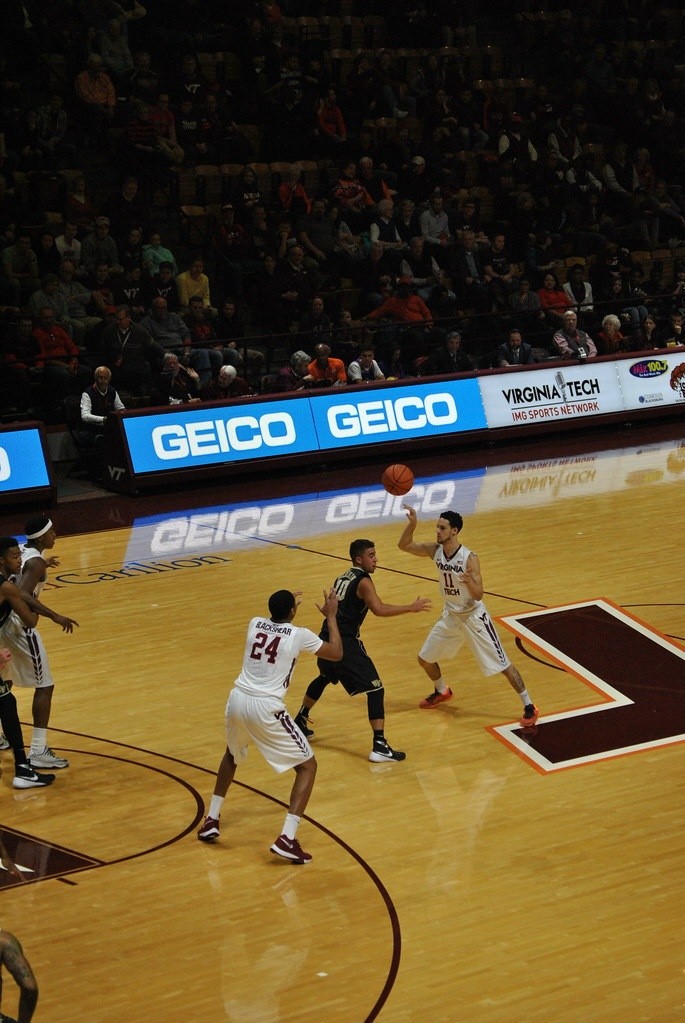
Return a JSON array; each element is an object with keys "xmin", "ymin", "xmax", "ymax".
[
  {"xmin": 0, "ymin": 0, "xmax": 685, "ymax": 482},
  {"xmin": 398, "ymin": 504, "xmax": 538, "ymax": 726},
  {"xmin": 294, "ymin": 539, "xmax": 433, "ymax": 763},
  {"xmin": 0, "ymin": 931, "xmax": 38, "ymax": 1023},
  {"xmin": 197, "ymin": 587, "xmax": 343, "ymax": 865},
  {"xmin": 0, "ymin": 517, "xmax": 79, "ymax": 789}
]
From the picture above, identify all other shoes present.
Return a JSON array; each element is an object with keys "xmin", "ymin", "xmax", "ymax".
[{"xmin": 0, "ymin": 732, "xmax": 10, "ymax": 750}]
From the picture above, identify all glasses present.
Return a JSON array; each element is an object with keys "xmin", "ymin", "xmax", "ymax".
[{"xmin": 97, "ymin": 377, "xmax": 110, "ymax": 383}]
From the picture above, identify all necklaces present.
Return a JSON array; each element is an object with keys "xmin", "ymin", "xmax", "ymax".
[{"xmin": 114, "ymin": 328, "xmax": 132, "ymax": 349}]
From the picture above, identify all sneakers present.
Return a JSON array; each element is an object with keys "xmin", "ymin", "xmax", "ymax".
[
  {"xmin": 269, "ymin": 835, "xmax": 313, "ymax": 865},
  {"xmin": 197, "ymin": 813, "xmax": 220, "ymax": 839},
  {"xmin": 368, "ymin": 737, "xmax": 407, "ymax": 763},
  {"xmin": 28, "ymin": 744, "xmax": 70, "ymax": 769},
  {"xmin": 520, "ymin": 703, "xmax": 539, "ymax": 725},
  {"xmin": 12, "ymin": 766, "xmax": 55, "ymax": 788},
  {"xmin": 419, "ymin": 684, "xmax": 455, "ymax": 709},
  {"xmin": 293, "ymin": 717, "xmax": 315, "ymax": 738}
]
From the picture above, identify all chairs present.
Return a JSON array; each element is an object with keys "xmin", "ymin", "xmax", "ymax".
[{"xmin": 0, "ymin": 0, "xmax": 685, "ymax": 479}]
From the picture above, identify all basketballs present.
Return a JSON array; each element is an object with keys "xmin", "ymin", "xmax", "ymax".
[{"xmin": 381, "ymin": 464, "xmax": 414, "ymax": 496}]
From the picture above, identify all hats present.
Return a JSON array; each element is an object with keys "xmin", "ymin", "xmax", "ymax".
[
  {"xmin": 313, "ymin": 344, "xmax": 333, "ymax": 356},
  {"xmin": 412, "ymin": 156, "xmax": 425, "ymax": 164},
  {"xmin": 94, "ymin": 216, "xmax": 110, "ymax": 226}
]
